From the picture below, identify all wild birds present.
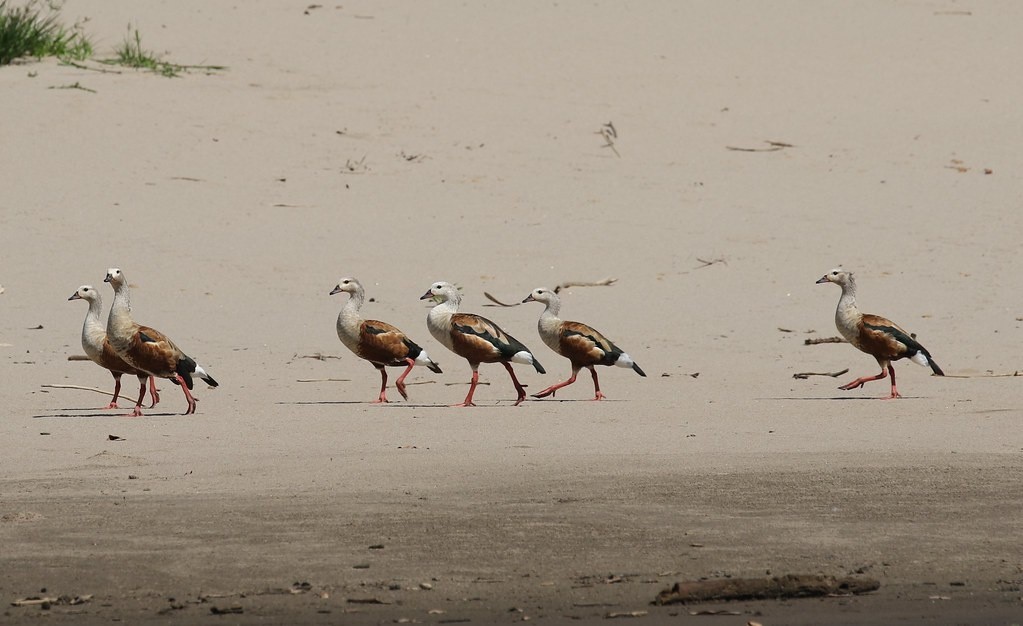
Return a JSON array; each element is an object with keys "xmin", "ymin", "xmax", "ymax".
[
  {"xmin": 329, "ymin": 277, "xmax": 443, "ymax": 404},
  {"xmin": 67, "ymin": 268, "xmax": 219, "ymax": 416},
  {"xmin": 522, "ymin": 288, "xmax": 646, "ymax": 401},
  {"xmin": 816, "ymin": 268, "xmax": 945, "ymax": 400},
  {"xmin": 419, "ymin": 281, "xmax": 547, "ymax": 408}
]
